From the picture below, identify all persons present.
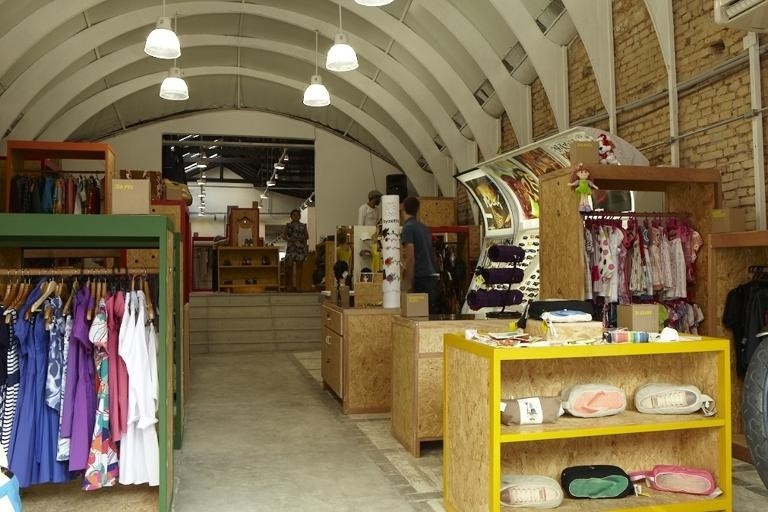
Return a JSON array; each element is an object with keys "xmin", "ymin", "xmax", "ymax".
[
  {"xmin": 567, "ymin": 168, "xmax": 598, "ymax": 212},
  {"xmin": 400, "ymin": 196, "xmax": 437, "ymax": 313},
  {"xmin": 357, "ymin": 189, "xmax": 383, "ymax": 226},
  {"xmin": 282, "ymin": 210, "xmax": 309, "ymax": 293}
]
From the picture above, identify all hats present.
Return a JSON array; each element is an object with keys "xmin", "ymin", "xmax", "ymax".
[{"xmin": 361, "ymin": 232, "xmax": 372, "ymax": 241}]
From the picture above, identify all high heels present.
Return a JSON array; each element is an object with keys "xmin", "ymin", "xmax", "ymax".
[
  {"xmin": 265, "ymin": 256, "xmax": 270, "ymax": 264},
  {"xmin": 249, "ymin": 238, "xmax": 254, "ymax": 247},
  {"xmin": 247, "ymin": 256, "xmax": 251, "ymax": 265},
  {"xmin": 242, "ymin": 256, "xmax": 247, "ymax": 265},
  {"xmin": 262, "ymin": 256, "xmax": 266, "ymax": 265},
  {"xmin": 244, "ymin": 238, "xmax": 249, "ymax": 247}
]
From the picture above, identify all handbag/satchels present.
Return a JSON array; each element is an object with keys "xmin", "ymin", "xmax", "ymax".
[
  {"xmin": 119, "ymin": 169, "xmax": 162, "ymax": 200},
  {"xmin": 526, "ymin": 298, "xmax": 596, "ymax": 322},
  {"xmin": 164, "ymin": 178, "xmax": 193, "ymax": 206},
  {"xmin": 359, "ymin": 240, "xmax": 372, "ymax": 257}
]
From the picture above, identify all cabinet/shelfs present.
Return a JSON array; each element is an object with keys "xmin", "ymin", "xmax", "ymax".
[
  {"xmin": 217, "ymin": 246, "xmax": 281, "ymax": 295},
  {"xmin": 226, "ymin": 206, "xmax": 260, "ymax": 246}
]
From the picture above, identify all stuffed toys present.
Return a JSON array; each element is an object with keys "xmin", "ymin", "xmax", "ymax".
[{"xmin": 595, "ymin": 134, "xmax": 619, "ymax": 165}]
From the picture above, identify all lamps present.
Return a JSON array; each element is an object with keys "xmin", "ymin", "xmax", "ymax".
[
  {"xmin": 157, "ymin": 16, "xmax": 191, "ymax": 103},
  {"xmin": 145, "ymin": 14, "xmax": 183, "ymax": 64},
  {"xmin": 303, "ymin": 28, "xmax": 331, "ymax": 110},
  {"xmin": 257, "ymin": 147, "xmax": 289, "ymax": 209},
  {"xmin": 325, "ymin": 0, "xmax": 359, "ymax": 73},
  {"xmin": 196, "ymin": 140, "xmax": 220, "ymax": 216}
]
